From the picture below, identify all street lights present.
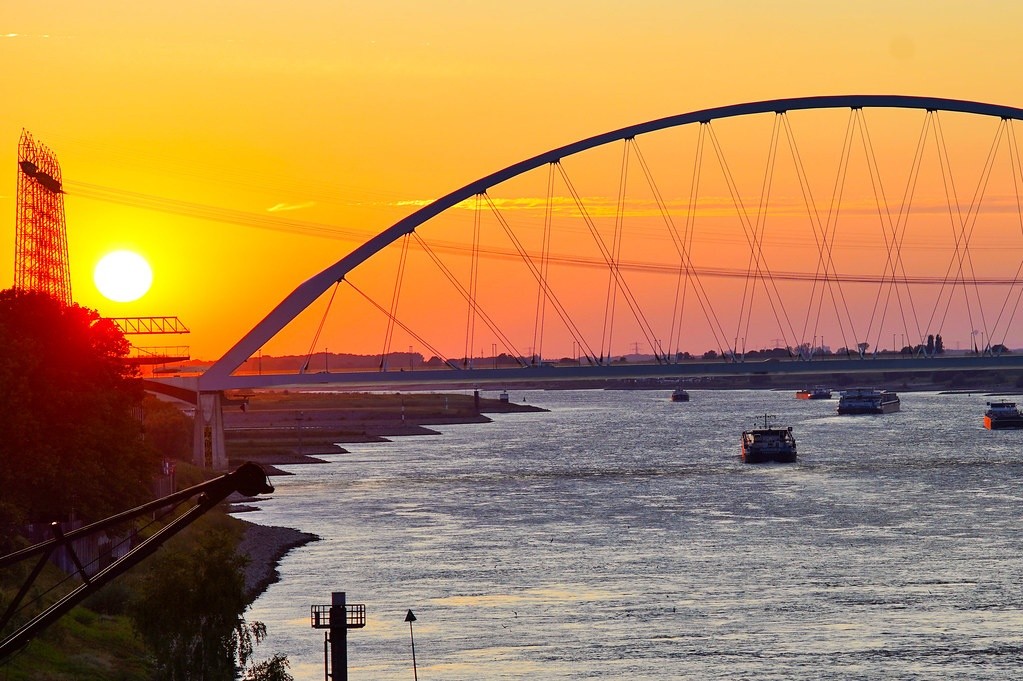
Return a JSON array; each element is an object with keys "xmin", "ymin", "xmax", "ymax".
[
  {"xmin": 970, "ymin": 332, "xmax": 973, "ymax": 354},
  {"xmin": 578, "ymin": 342, "xmax": 580, "ymax": 367},
  {"xmin": 734, "ymin": 337, "xmax": 737, "ymax": 357},
  {"xmin": 901, "ymin": 334, "xmax": 904, "ymax": 359},
  {"xmin": 659, "ymin": 340, "xmax": 661, "ymax": 364},
  {"xmin": 409, "ymin": 346, "xmax": 412, "ymax": 372},
  {"xmin": 258, "ymin": 349, "xmax": 261, "ymax": 375},
  {"xmin": 654, "ymin": 340, "xmax": 656, "ymax": 364},
  {"xmin": 573, "ymin": 342, "xmax": 575, "ymax": 366},
  {"xmin": 893, "ymin": 333, "xmax": 895, "ymax": 359},
  {"xmin": 741, "ymin": 338, "xmax": 744, "ymax": 362},
  {"xmin": 491, "ymin": 344, "xmax": 494, "ymax": 369},
  {"xmin": 494, "ymin": 344, "xmax": 497, "ymax": 369},
  {"xmin": 814, "ymin": 336, "xmax": 817, "ymax": 362},
  {"xmin": 980, "ymin": 332, "xmax": 984, "ymax": 356},
  {"xmin": 820, "ymin": 336, "xmax": 823, "ymax": 361},
  {"xmin": 325, "ymin": 348, "xmax": 328, "ymax": 372}
]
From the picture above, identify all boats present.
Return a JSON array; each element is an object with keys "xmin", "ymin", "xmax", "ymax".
[
  {"xmin": 795, "ymin": 384, "xmax": 832, "ymax": 400},
  {"xmin": 740, "ymin": 413, "xmax": 796, "ymax": 464},
  {"xmin": 671, "ymin": 388, "xmax": 689, "ymax": 402},
  {"xmin": 837, "ymin": 386, "xmax": 900, "ymax": 415},
  {"xmin": 983, "ymin": 399, "xmax": 1023, "ymax": 431}
]
